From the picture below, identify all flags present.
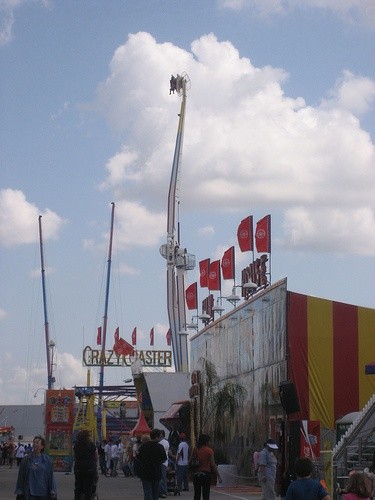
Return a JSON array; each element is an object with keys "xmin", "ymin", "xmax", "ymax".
[
  {"xmin": 199, "ymin": 258, "xmax": 221, "ymax": 290},
  {"xmin": 114, "ymin": 326, "xmax": 119, "ymax": 344},
  {"xmin": 221, "ymin": 246, "xmax": 235, "ymax": 279},
  {"xmin": 149, "ymin": 328, "xmax": 154, "ymax": 345},
  {"xmin": 255, "ymin": 215, "xmax": 270, "ymax": 252},
  {"xmin": 166, "ymin": 328, "xmax": 171, "ymax": 346},
  {"xmin": 97, "ymin": 327, "xmax": 101, "ymax": 345},
  {"xmin": 237, "ymin": 216, "xmax": 254, "ymax": 252},
  {"xmin": 185, "ymin": 282, "xmax": 197, "ymax": 309},
  {"xmin": 113, "ymin": 337, "xmax": 134, "ymax": 355},
  {"xmin": 132, "ymin": 327, "xmax": 136, "ymax": 345}
]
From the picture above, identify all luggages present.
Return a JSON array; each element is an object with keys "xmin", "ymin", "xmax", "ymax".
[
  {"xmin": 105, "ymin": 457, "xmax": 112, "ymax": 477},
  {"xmin": 122, "ymin": 461, "xmax": 135, "ymax": 477}
]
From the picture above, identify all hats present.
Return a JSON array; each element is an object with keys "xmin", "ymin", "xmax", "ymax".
[
  {"xmin": 266, "ymin": 443, "xmax": 279, "ymax": 449},
  {"xmin": 179, "ymin": 432, "xmax": 186, "ymax": 440}
]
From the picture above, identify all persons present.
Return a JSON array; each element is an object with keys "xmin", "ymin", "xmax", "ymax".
[
  {"xmin": 158, "ymin": 430, "xmax": 170, "ymax": 497},
  {"xmin": 73, "ymin": 430, "xmax": 100, "ymax": 500},
  {"xmin": 138, "ymin": 429, "xmax": 168, "ymax": 500},
  {"xmin": 96, "ymin": 433, "xmax": 148, "ymax": 478},
  {"xmin": 15, "ymin": 437, "xmax": 58, "ymax": 500},
  {"xmin": 189, "ymin": 434, "xmax": 222, "ymax": 500},
  {"xmin": 343, "ymin": 467, "xmax": 375, "ymax": 500},
  {"xmin": 283, "ymin": 457, "xmax": 330, "ymax": 500},
  {"xmin": 176, "ymin": 433, "xmax": 189, "ymax": 491},
  {"xmin": 256, "ymin": 439, "xmax": 278, "ymax": 500},
  {"xmin": 0, "ymin": 443, "xmax": 33, "ymax": 469}
]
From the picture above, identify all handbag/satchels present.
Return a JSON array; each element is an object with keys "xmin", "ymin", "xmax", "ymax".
[
  {"xmin": 189, "ymin": 447, "xmax": 203, "ymax": 476},
  {"xmin": 91, "ymin": 483, "xmax": 99, "ymax": 500},
  {"xmin": 133, "ymin": 449, "xmax": 146, "ymax": 480}
]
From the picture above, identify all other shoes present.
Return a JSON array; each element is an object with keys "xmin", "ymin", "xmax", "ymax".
[
  {"xmin": 180, "ymin": 487, "xmax": 189, "ymax": 492},
  {"xmin": 161, "ymin": 495, "xmax": 167, "ymax": 498}
]
